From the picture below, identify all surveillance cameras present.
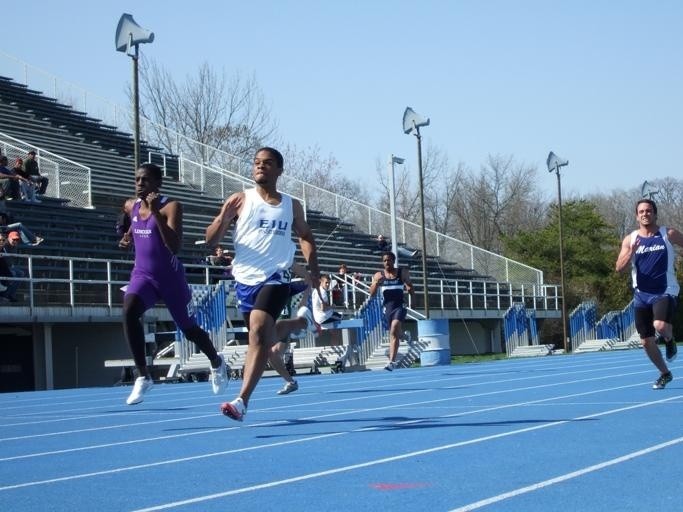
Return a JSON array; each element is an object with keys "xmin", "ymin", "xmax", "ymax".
[{"xmin": 393, "ymin": 156, "xmax": 404, "ymax": 164}]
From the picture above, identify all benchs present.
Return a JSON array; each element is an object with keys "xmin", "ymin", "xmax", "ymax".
[{"xmin": 0, "ymin": 72, "xmax": 545, "ymax": 310}]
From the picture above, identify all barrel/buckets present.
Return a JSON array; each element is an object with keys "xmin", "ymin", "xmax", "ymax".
[{"xmin": 417, "ymin": 319, "xmax": 451, "ymax": 367}]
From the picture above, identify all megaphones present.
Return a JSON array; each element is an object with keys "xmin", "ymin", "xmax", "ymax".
[
  {"xmin": 115, "ymin": 13, "xmax": 154, "ymax": 52},
  {"xmin": 642, "ymin": 180, "xmax": 658, "ymax": 197},
  {"xmin": 403, "ymin": 106, "xmax": 430, "ymax": 134},
  {"xmin": 547, "ymin": 151, "xmax": 568, "ymax": 172}
]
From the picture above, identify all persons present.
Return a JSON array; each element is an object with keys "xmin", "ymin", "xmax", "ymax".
[
  {"xmin": 370, "ymin": 251, "xmax": 414, "ymax": 371},
  {"xmin": 312, "ymin": 274, "xmax": 343, "ymax": 346},
  {"xmin": 0, "ymin": 150, "xmax": 49, "ymax": 215},
  {"xmin": 616, "ymin": 200, "xmax": 683, "ymax": 390},
  {"xmin": 210, "ymin": 245, "xmax": 234, "ymax": 284},
  {"xmin": 268, "ymin": 297, "xmax": 298, "ymax": 395},
  {"xmin": 373, "ymin": 234, "xmax": 418, "ymax": 258},
  {"xmin": 206, "ymin": 148, "xmax": 322, "ymax": 422},
  {"xmin": 117, "ymin": 163, "xmax": 230, "ymax": 404},
  {"xmin": 0, "ymin": 234, "xmax": 12, "ymax": 295},
  {"xmin": 346, "ymin": 271, "xmax": 361, "ymax": 305},
  {"xmin": 333, "ymin": 264, "xmax": 349, "ymax": 309},
  {"xmin": 3, "ymin": 231, "xmax": 25, "ymax": 301},
  {"xmin": 0, "ymin": 212, "xmax": 44, "ymax": 247},
  {"xmin": 114, "ymin": 204, "xmax": 131, "ymax": 239}
]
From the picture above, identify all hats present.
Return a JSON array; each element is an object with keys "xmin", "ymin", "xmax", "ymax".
[{"xmin": 9, "ymin": 231, "xmax": 20, "ymax": 239}]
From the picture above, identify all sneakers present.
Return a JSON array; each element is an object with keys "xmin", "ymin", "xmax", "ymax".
[
  {"xmin": 127, "ymin": 376, "xmax": 153, "ymax": 405},
  {"xmin": 653, "ymin": 371, "xmax": 672, "ymax": 389},
  {"xmin": 210, "ymin": 354, "xmax": 246, "ymax": 421},
  {"xmin": 666, "ymin": 337, "xmax": 677, "ymax": 361},
  {"xmin": 277, "ymin": 381, "xmax": 298, "ymax": 394},
  {"xmin": 385, "ymin": 361, "xmax": 395, "ymax": 371}
]
